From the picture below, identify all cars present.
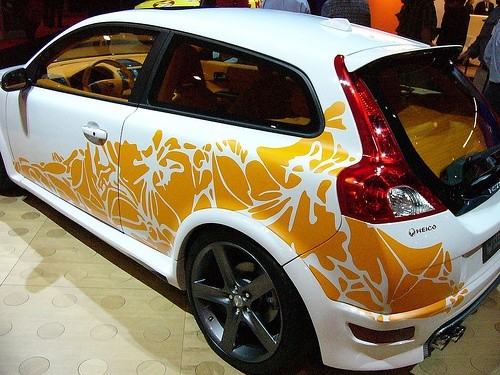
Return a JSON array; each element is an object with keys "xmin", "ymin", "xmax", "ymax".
[{"xmin": 0, "ymin": 6, "xmax": 500, "ymax": 374}]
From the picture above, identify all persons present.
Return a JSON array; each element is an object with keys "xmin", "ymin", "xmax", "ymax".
[
  {"xmin": 474, "ymin": 0, "xmax": 494, "ymax": 16},
  {"xmin": 483, "ymin": 19, "xmax": 500, "ymax": 110},
  {"xmin": 395, "ymin": 0, "xmax": 437, "ymax": 46},
  {"xmin": 2, "ymin": 0, "xmax": 65, "ymax": 46},
  {"xmin": 320, "ymin": 0, "xmax": 371, "ymax": 27},
  {"xmin": 453, "ymin": 0, "xmax": 500, "ymax": 96},
  {"xmin": 261, "ymin": 0, "xmax": 311, "ymax": 14},
  {"xmin": 462, "ymin": 0, "xmax": 474, "ymax": 13},
  {"xmin": 436, "ymin": 0, "xmax": 470, "ymax": 46}
]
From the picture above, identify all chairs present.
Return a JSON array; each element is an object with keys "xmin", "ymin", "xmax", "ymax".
[
  {"xmin": 158, "ymin": 44, "xmax": 218, "ymax": 110},
  {"xmin": 231, "ymin": 56, "xmax": 290, "ymax": 123}
]
